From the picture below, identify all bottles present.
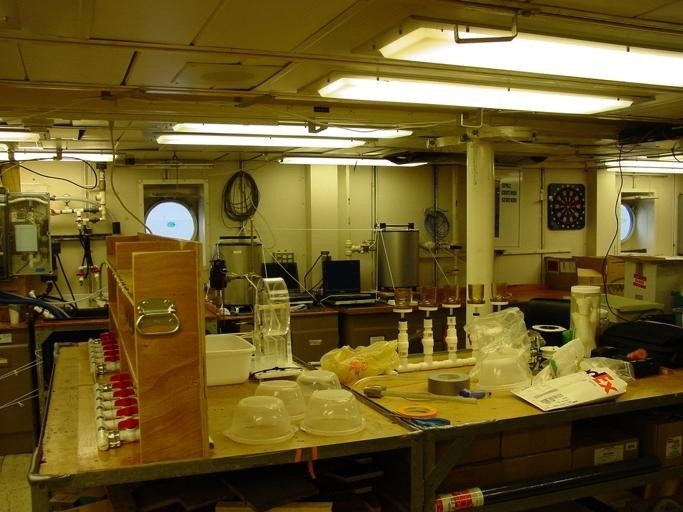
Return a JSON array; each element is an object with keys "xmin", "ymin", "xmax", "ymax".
[{"xmin": 569, "ymin": 284, "xmax": 602, "ymax": 351}]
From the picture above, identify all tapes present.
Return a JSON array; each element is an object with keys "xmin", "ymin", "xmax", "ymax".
[
  {"xmin": 428, "ymin": 372, "xmax": 470, "ymax": 395},
  {"xmin": 395, "ymin": 404, "xmax": 437, "ymax": 419}
]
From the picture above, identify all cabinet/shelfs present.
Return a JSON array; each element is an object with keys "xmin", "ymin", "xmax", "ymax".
[
  {"xmin": 104, "ymin": 231, "xmax": 209, "ymax": 465},
  {"xmin": 0, "ymin": 343, "xmax": 36, "ymax": 456}
]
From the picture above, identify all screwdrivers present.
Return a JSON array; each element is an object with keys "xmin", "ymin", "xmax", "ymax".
[{"xmin": 387, "ymin": 414, "xmax": 450, "ymax": 426}]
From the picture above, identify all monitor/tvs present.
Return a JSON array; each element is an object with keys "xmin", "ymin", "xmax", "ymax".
[
  {"xmin": 262, "ymin": 262, "xmax": 300, "ymax": 293},
  {"xmin": 322, "ymin": 260, "xmax": 360, "ymax": 294}
]
